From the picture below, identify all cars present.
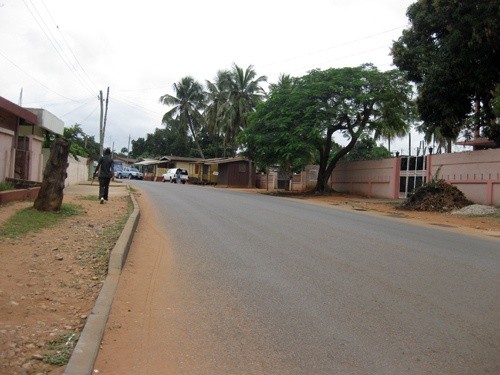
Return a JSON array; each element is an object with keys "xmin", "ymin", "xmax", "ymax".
[
  {"xmin": 121, "ymin": 167, "xmax": 144, "ymax": 180},
  {"xmin": 93, "ymin": 159, "xmax": 122, "ymax": 176},
  {"xmin": 162, "ymin": 168, "xmax": 189, "ymax": 184}
]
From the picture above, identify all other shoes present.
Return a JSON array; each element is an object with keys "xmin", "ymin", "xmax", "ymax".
[{"xmin": 100, "ymin": 197, "xmax": 108, "ymax": 204}]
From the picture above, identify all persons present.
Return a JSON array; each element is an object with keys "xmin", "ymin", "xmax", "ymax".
[
  {"xmin": 176, "ymin": 170, "xmax": 181, "ymax": 184},
  {"xmin": 93, "ymin": 148, "xmax": 115, "ymax": 203}
]
